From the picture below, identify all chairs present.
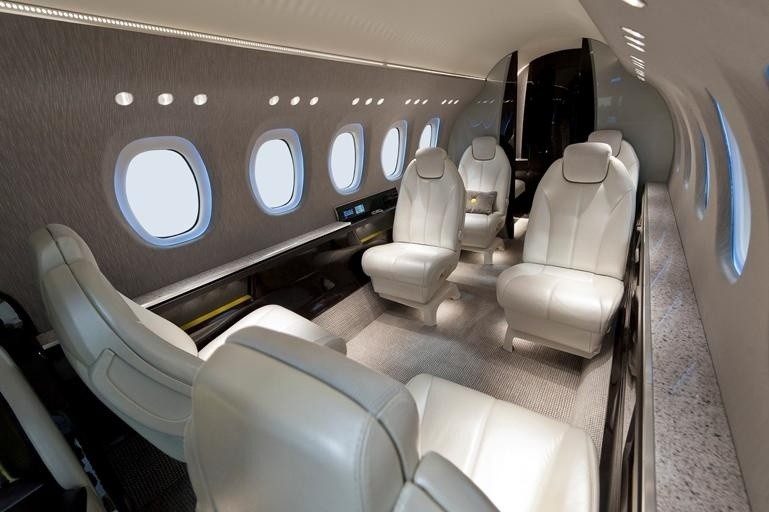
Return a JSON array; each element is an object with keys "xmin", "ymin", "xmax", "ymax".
[
  {"xmin": 182, "ymin": 325, "xmax": 600, "ymax": 509},
  {"xmin": 32, "ymin": 223, "xmax": 352, "ymax": 467},
  {"xmin": 581, "ymin": 129, "xmax": 640, "ymax": 190},
  {"xmin": 360, "ymin": 148, "xmax": 468, "ymax": 327},
  {"xmin": 456, "ymin": 136, "xmax": 510, "ymax": 264},
  {"xmin": 497, "ymin": 143, "xmax": 635, "ymax": 358},
  {"xmin": 514, "ymin": 179, "xmax": 525, "ymax": 198}
]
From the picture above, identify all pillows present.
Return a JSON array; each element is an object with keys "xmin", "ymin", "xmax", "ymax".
[{"xmin": 465, "ymin": 190, "xmax": 497, "ymax": 215}]
